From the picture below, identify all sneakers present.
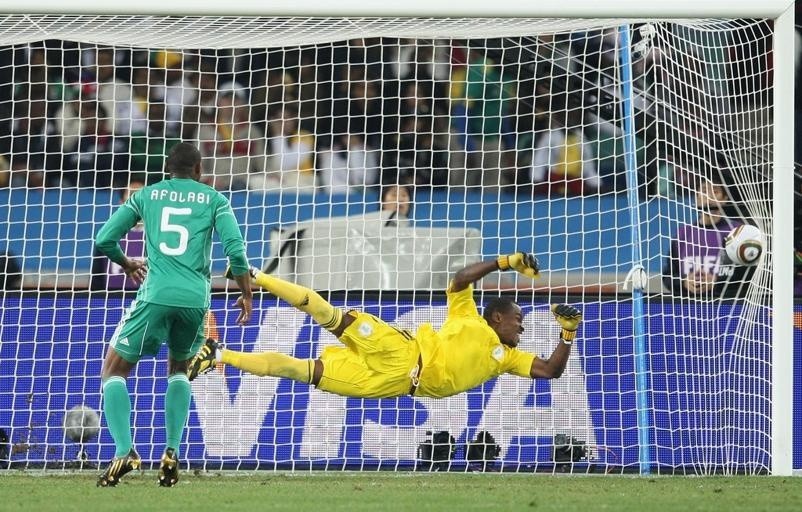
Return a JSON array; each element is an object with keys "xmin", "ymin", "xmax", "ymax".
[
  {"xmin": 159, "ymin": 446, "xmax": 179, "ymax": 487},
  {"xmin": 225, "ymin": 262, "xmax": 252, "ymax": 280},
  {"xmin": 97, "ymin": 452, "xmax": 141, "ymax": 487},
  {"xmin": 187, "ymin": 338, "xmax": 225, "ymax": 378}
]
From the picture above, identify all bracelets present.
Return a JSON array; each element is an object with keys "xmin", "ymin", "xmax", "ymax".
[{"xmin": 561, "ymin": 338, "xmax": 571, "ymax": 345}]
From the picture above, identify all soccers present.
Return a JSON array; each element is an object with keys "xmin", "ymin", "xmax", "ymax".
[
  {"xmin": 62, "ymin": 406, "xmax": 100, "ymax": 443},
  {"xmin": 725, "ymin": 225, "xmax": 763, "ymax": 265}
]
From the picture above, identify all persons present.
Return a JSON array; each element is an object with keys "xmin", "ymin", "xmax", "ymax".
[
  {"xmin": 185, "ymin": 252, "xmax": 583, "ymax": 399},
  {"xmin": 382, "ymin": 186, "xmax": 412, "ymax": 216},
  {"xmin": 2, "ymin": 18, "xmax": 603, "ymax": 192},
  {"xmin": 664, "ymin": 180, "xmax": 746, "ymax": 298},
  {"xmin": 96, "ymin": 142, "xmax": 253, "ymax": 487},
  {"xmin": 92, "ymin": 181, "xmax": 144, "ymax": 290}
]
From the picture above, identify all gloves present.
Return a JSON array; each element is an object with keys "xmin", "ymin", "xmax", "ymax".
[
  {"xmin": 499, "ymin": 254, "xmax": 541, "ymax": 277},
  {"xmin": 550, "ymin": 304, "xmax": 582, "ymax": 343}
]
